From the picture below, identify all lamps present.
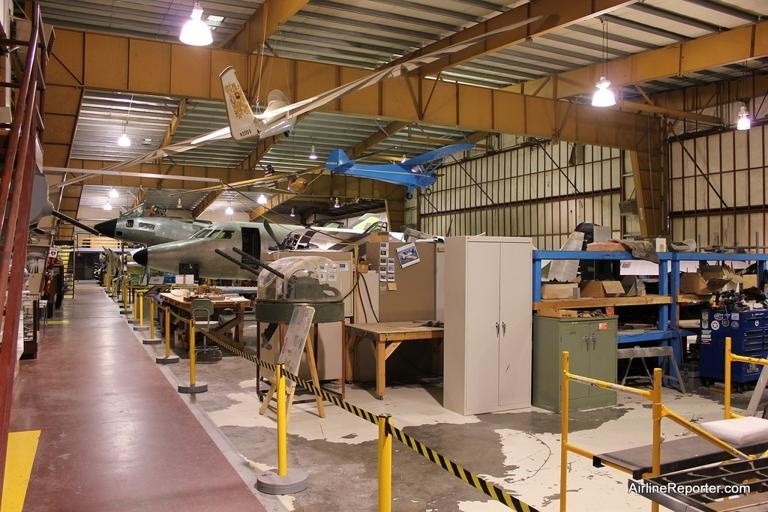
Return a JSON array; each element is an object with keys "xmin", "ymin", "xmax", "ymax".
[
  {"xmin": 175, "ymin": 196, "xmax": 183, "ymax": 208},
  {"xmin": 736, "ymin": 62, "xmax": 750, "ymax": 131},
  {"xmin": 411, "ymin": 165, "xmax": 420, "ymax": 173},
  {"xmin": 225, "ymin": 193, "xmax": 342, "ymax": 217},
  {"xmin": 591, "ymin": 17, "xmax": 616, "ymax": 107},
  {"xmin": 102, "ymin": 185, "xmax": 120, "ymax": 213},
  {"xmin": 309, "ymin": 146, "xmax": 318, "ymax": 160},
  {"xmin": 118, "ymin": 119, "xmax": 130, "ymax": 146},
  {"xmin": 179, "ymin": 0, "xmax": 213, "ymax": 47}
]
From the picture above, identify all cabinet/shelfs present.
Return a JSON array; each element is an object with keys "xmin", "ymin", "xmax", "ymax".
[
  {"xmin": 533, "ymin": 250, "xmax": 767, "ymax": 386},
  {"xmin": 443, "ymin": 236, "xmax": 533, "ymax": 416},
  {"xmin": 533, "ymin": 315, "xmax": 618, "ymax": 413}
]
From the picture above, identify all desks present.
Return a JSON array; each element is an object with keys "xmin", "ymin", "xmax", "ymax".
[
  {"xmin": 346, "ymin": 322, "xmax": 444, "ymax": 401},
  {"xmin": 159, "ymin": 293, "xmax": 251, "ymax": 351}
]
